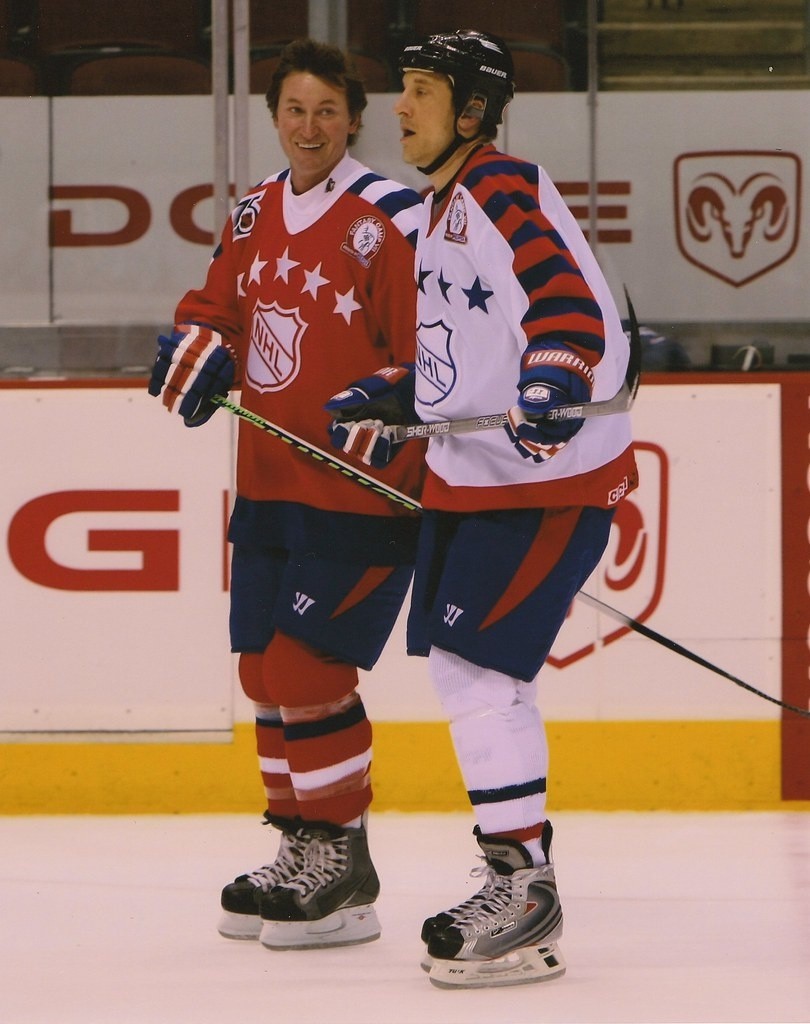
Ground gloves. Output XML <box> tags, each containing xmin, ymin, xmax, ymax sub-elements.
<box><xmin>323</xmin><ymin>363</ymin><xmax>422</xmax><ymax>469</ymax></box>
<box><xmin>504</xmin><ymin>341</ymin><xmax>594</xmax><ymax>463</ymax></box>
<box><xmin>147</xmin><ymin>321</ymin><xmax>236</xmax><ymax>427</ymax></box>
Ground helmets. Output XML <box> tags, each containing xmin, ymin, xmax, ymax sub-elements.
<box><xmin>398</xmin><ymin>28</ymin><xmax>515</xmax><ymax>142</ymax></box>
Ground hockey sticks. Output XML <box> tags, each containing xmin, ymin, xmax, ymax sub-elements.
<box><xmin>216</xmin><ymin>391</ymin><xmax>810</xmax><ymax>717</ymax></box>
<box><xmin>382</xmin><ymin>279</ymin><xmax>643</xmax><ymax>445</ymax></box>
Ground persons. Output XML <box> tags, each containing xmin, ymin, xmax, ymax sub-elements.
<box><xmin>149</xmin><ymin>42</ymin><xmax>422</xmax><ymax>950</ymax></box>
<box><xmin>322</xmin><ymin>28</ymin><xmax>639</xmax><ymax>987</ymax></box>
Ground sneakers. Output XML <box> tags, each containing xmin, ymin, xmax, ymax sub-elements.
<box><xmin>427</xmin><ymin>826</ymin><xmax>566</xmax><ymax>985</ymax></box>
<box><xmin>420</xmin><ymin>818</ymin><xmax>552</xmax><ymax>971</ymax></box>
<box><xmin>215</xmin><ymin>809</ymin><xmax>304</xmax><ymax>935</ymax></box>
<box><xmin>260</xmin><ymin>814</ymin><xmax>381</xmax><ymax>946</ymax></box>
